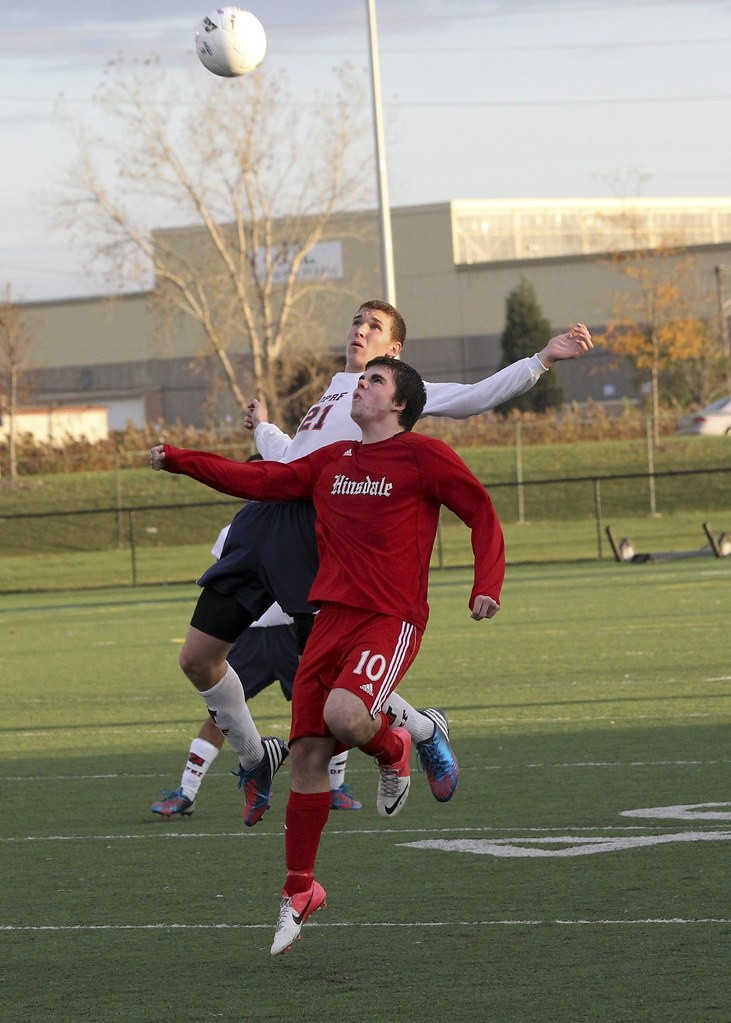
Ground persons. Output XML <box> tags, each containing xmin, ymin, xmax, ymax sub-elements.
<box><xmin>147</xmin><ymin>354</ymin><xmax>504</xmax><ymax>953</ymax></box>
<box><xmin>148</xmin><ymin>295</ymin><xmax>594</xmax><ymax>825</ymax></box>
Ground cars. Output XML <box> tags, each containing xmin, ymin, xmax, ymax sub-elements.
<box><xmin>679</xmin><ymin>397</ymin><xmax>731</xmax><ymax>437</ymax></box>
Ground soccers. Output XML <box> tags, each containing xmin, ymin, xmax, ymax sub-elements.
<box><xmin>195</xmin><ymin>6</ymin><xmax>267</xmax><ymax>79</ymax></box>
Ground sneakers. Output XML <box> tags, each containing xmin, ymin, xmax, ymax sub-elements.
<box><xmin>415</xmin><ymin>707</ymin><xmax>458</xmax><ymax>802</ymax></box>
<box><xmin>270</xmin><ymin>880</ymin><xmax>327</xmax><ymax>956</ymax></box>
<box><xmin>373</xmin><ymin>727</ymin><xmax>411</xmax><ymax>818</ymax></box>
<box><xmin>230</xmin><ymin>736</ymin><xmax>289</xmax><ymax>827</ymax></box>
<box><xmin>151</xmin><ymin>787</ymin><xmax>196</xmax><ymax>818</ymax></box>
<box><xmin>329</xmin><ymin>784</ymin><xmax>362</xmax><ymax>810</ymax></box>
